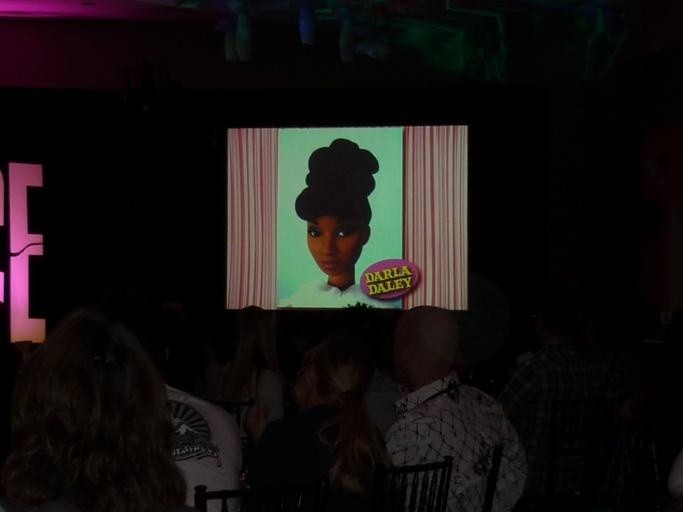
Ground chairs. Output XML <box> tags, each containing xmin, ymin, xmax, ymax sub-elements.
<box><xmin>194</xmin><ymin>398</ymin><xmax>683</xmax><ymax>512</ymax></box>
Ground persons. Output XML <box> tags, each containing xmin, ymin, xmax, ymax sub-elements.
<box><xmin>1</xmin><ymin>306</ymin><xmax>682</xmax><ymax>511</ymax></box>
<box><xmin>278</xmin><ymin>138</ymin><xmax>393</xmax><ymax>308</ymax></box>
